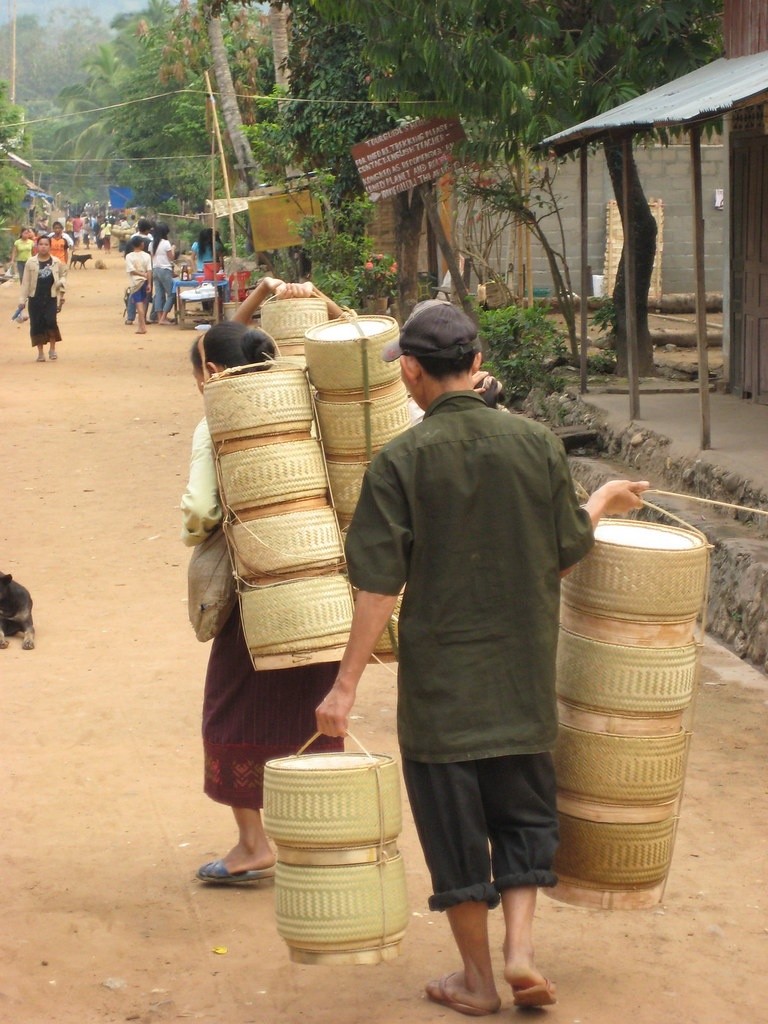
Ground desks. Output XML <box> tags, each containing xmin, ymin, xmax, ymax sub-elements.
<box><xmin>171</xmin><ymin>275</ymin><xmax>229</xmax><ymax>325</ymax></box>
<box><xmin>178</xmin><ymin>291</ymin><xmax>220</xmax><ymax>328</ymax></box>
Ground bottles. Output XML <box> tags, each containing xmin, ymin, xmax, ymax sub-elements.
<box><xmin>181</xmin><ymin>265</ymin><xmax>188</xmax><ymax>281</ymax></box>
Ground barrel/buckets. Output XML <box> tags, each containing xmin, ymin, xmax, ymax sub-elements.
<box><xmin>223</xmin><ymin>303</ymin><xmax>242</xmax><ymax>322</ymax></box>
<box><xmin>201</xmin><ymin>280</ymin><xmax>231</xmax><ymax>314</ymax></box>
<box><xmin>592</xmin><ymin>274</ymin><xmax>604</xmax><ymax>298</ymax></box>
<box><xmin>203</xmin><ymin>261</ymin><xmax>219</xmax><ymax>281</ymax></box>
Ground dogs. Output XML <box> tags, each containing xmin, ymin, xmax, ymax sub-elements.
<box><xmin>69</xmin><ymin>249</ymin><xmax>92</xmax><ymax>270</ymax></box>
<box><xmin>0</xmin><ymin>572</ymin><xmax>37</xmax><ymax>651</ymax></box>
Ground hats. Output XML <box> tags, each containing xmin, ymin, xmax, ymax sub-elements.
<box><xmin>380</xmin><ymin>296</ymin><xmax>482</xmax><ymax>359</ymax></box>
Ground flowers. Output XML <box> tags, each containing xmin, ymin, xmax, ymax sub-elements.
<box><xmin>358</xmin><ymin>252</ymin><xmax>396</xmax><ymax>301</ymax></box>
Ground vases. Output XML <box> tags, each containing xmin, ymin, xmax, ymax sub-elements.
<box><xmin>363</xmin><ymin>294</ymin><xmax>389</xmax><ymax>316</ymax></box>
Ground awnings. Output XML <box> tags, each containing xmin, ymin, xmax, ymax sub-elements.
<box><xmin>539</xmin><ymin>51</ymin><xmax>768</xmax><ymax>450</ymax></box>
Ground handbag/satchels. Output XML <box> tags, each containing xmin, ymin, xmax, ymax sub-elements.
<box><xmin>5</xmin><ymin>265</ymin><xmax>12</xmax><ymax>278</ymax></box>
<box><xmin>186</xmin><ymin>522</ymin><xmax>241</xmax><ymax>643</ymax></box>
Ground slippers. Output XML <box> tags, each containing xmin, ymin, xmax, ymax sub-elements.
<box><xmin>125</xmin><ymin>320</ymin><xmax>133</xmax><ymax>325</ymax></box>
<box><xmin>426</xmin><ymin>970</ymin><xmax>493</xmax><ymax>1015</ymax></box>
<box><xmin>511</xmin><ymin>971</ymin><xmax>556</xmax><ymax>1009</ymax></box>
<box><xmin>37</xmin><ymin>355</ymin><xmax>46</xmax><ymax>361</ymax></box>
<box><xmin>196</xmin><ymin>858</ymin><xmax>276</xmax><ymax>883</ymax></box>
<box><xmin>49</xmin><ymin>350</ymin><xmax>57</xmax><ymax>360</ymax></box>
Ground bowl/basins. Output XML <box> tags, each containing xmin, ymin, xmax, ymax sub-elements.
<box><xmin>195</xmin><ymin>276</ymin><xmax>204</xmax><ymax>281</ymax></box>
<box><xmin>216</xmin><ymin>273</ymin><xmax>224</xmax><ymax>280</ymax></box>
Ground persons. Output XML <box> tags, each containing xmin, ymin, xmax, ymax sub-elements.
<box><xmin>191</xmin><ymin>228</ymin><xmax>224</xmax><ymax>312</ymax></box>
<box><xmin>181</xmin><ymin>322</ymin><xmax>344</xmax><ymax>884</ymax></box>
<box><xmin>18</xmin><ymin>236</ymin><xmax>66</xmax><ymax>361</ymax></box>
<box><xmin>407</xmin><ymin>370</ymin><xmax>504</xmax><ymax>426</ymax></box>
<box><xmin>124</xmin><ymin>220</ymin><xmax>176</xmax><ymax>334</ymax></box>
<box><xmin>11</xmin><ymin>212</ymin><xmax>69</xmax><ymax>313</ymax></box>
<box><xmin>66</xmin><ymin>201</ymin><xmax>136</xmax><ymax>255</ymax></box>
<box><xmin>232</xmin><ymin>276</ymin><xmax>342</xmax><ymax>319</ymax></box>
<box><xmin>316</xmin><ymin>303</ymin><xmax>650</xmax><ymax>1017</ymax></box>
